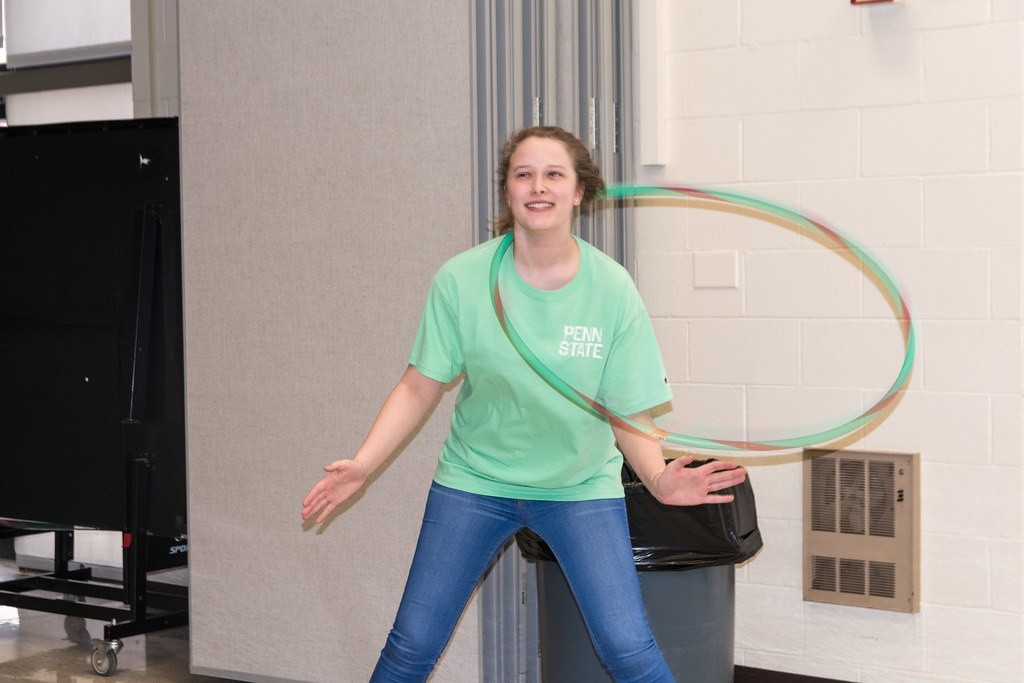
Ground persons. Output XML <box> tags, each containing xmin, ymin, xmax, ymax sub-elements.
<box><xmin>300</xmin><ymin>122</ymin><xmax>748</xmax><ymax>683</ymax></box>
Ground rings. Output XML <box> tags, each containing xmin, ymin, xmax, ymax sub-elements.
<box><xmin>707</xmin><ymin>484</ymin><xmax>711</xmax><ymax>493</ymax></box>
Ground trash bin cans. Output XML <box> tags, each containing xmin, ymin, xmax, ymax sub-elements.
<box><xmin>515</xmin><ymin>458</ymin><xmax>763</xmax><ymax>683</ymax></box>
